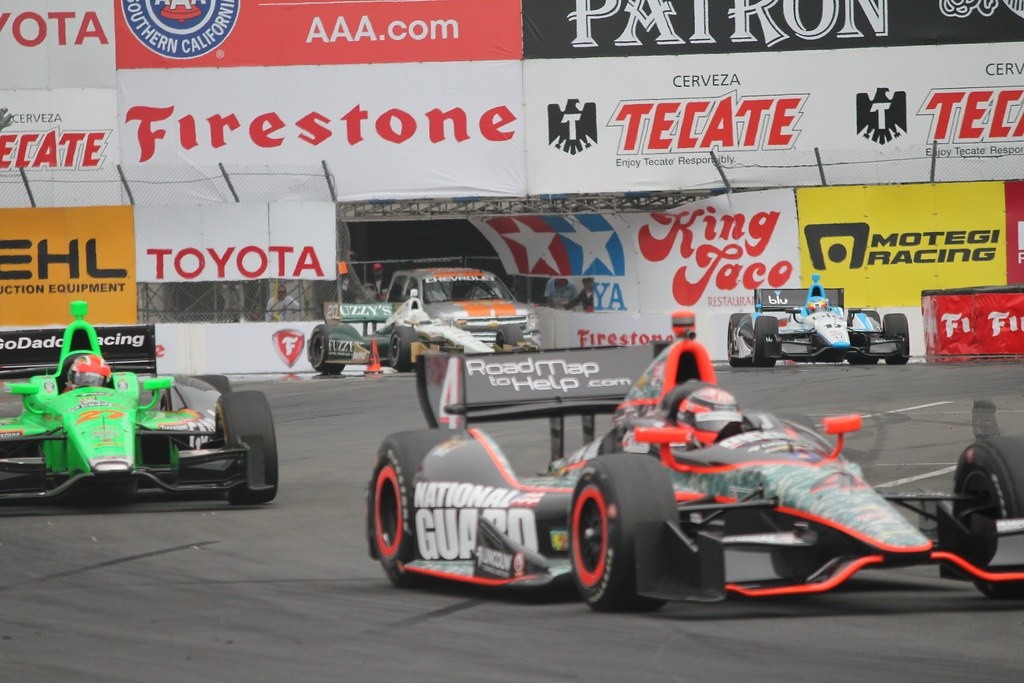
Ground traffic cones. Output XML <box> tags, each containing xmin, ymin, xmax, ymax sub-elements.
<box><xmin>363</xmin><ymin>337</ymin><xmax>388</xmax><ymax>376</ymax></box>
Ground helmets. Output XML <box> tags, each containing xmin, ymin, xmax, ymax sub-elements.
<box><xmin>806</xmin><ymin>295</ymin><xmax>828</xmax><ymax>316</ymax></box>
<box><xmin>66</xmin><ymin>355</ymin><xmax>111</xmax><ymax>391</ymax></box>
<box><xmin>676</xmin><ymin>388</ymin><xmax>742</xmax><ymax>450</ymax></box>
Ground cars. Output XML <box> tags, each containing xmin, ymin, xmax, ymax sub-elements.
<box><xmin>382</xmin><ymin>267</ymin><xmax>540</xmax><ymax>358</ymax></box>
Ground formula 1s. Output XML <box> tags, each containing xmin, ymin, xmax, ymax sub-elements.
<box><xmin>727</xmin><ymin>271</ymin><xmax>912</xmax><ymax>367</ymax></box>
<box><xmin>0</xmin><ymin>300</ymin><xmax>279</xmax><ymax>507</ymax></box>
<box><xmin>306</xmin><ymin>290</ymin><xmax>494</xmax><ymax>379</ymax></box>
<box><xmin>366</xmin><ymin>314</ymin><xmax>1023</xmax><ymax>617</ymax></box>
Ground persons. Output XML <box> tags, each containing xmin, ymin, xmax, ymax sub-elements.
<box><xmin>676</xmin><ymin>387</ymin><xmax>738</xmax><ymax>448</ymax></box>
<box><xmin>265</xmin><ymin>286</ymin><xmax>302</xmax><ymax>322</ymax></box>
<box><xmin>565</xmin><ymin>277</ymin><xmax>594</xmax><ymax>311</ymax></box>
<box><xmin>544</xmin><ymin>277</ymin><xmax>576</xmax><ymax>310</ymax></box>
<box><xmin>65</xmin><ymin>354</ymin><xmax>111</xmax><ymax>392</ymax></box>
<box><xmin>808</xmin><ymin>296</ymin><xmax>827</xmax><ymax>313</ymax></box>
<box><xmin>370</xmin><ymin>263</ymin><xmax>389</xmax><ymax>302</ymax></box>
